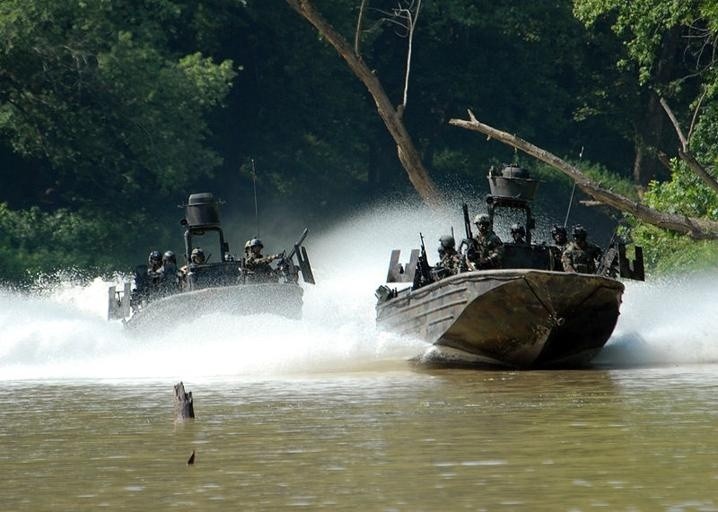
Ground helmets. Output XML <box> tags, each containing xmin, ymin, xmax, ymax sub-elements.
<box><xmin>250</xmin><ymin>239</ymin><xmax>263</xmax><ymax>248</ymax></box>
<box><xmin>245</xmin><ymin>239</ymin><xmax>251</xmax><ymax>248</ymax></box>
<box><xmin>438</xmin><ymin>235</ymin><xmax>455</xmax><ymax>249</ymax></box>
<box><xmin>510</xmin><ymin>223</ymin><xmax>526</xmax><ymax>234</ymax></box>
<box><xmin>472</xmin><ymin>213</ymin><xmax>491</xmax><ymax>225</ymax></box>
<box><xmin>149</xmin><ymin>250</ymin><xmax>162</xmax><ymax>260</ymax></box>
<box><xmin>572</xmin><ymin>226</ymin><xmax>587</xmax><ymax>235</ymax></box>
<box><xmin>161</xmin><ymin>250</ymin><xmax>177</xmax><ymax>262</ymax></box>
<box><xmin>551</xmin><ymin>225</ymin><xmax>566</xmax><ymax>236</ymax></box>
<box><xmin>191</xmin><ymin>248</ymin><xmax>205</xmax><ymax>258</ymax></box>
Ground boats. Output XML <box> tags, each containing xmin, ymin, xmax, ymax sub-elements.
<box><xmin>107</xmin><ymin>159</ymin><xmax>316</xmax><ymax>369</ymax></box>
<box><xmin>372</xmin><ymin>146</ymin><xmax>646</xmax><ymax>374</ymax></box>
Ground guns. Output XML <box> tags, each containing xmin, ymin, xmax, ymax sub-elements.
<box><xmin>451</xmin><ymin>226</ymin><xmax>456</xmax><ymax>249</ymax></box>
<box><xmin>418</xmin><ymin>232</ymin><xmax>430</xmax><ymax>276</ymax></box>
<box><xmin>462</xmin><ymin>202</ymin><xmax>474</xmax><ymax>240</ymax></box>
<box><xmin>596</xmin><ymin>232</ymin><xmax>617</xmax><ymax>274</ymax></box>
<box><xmin>278</xmin><ymin>227</ymin><xmax>309</xmax><ymax>267</ymax></box>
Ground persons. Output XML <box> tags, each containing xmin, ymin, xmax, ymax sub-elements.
<box><xmin>148</xmin><ymin>237</ymin><xmax>283</xmax><ymax>295</ymax></box>
<box><xmin>430</xmin><ymin>213</ymin><xmax>606</xmax><ymax>281</ymax></box>
